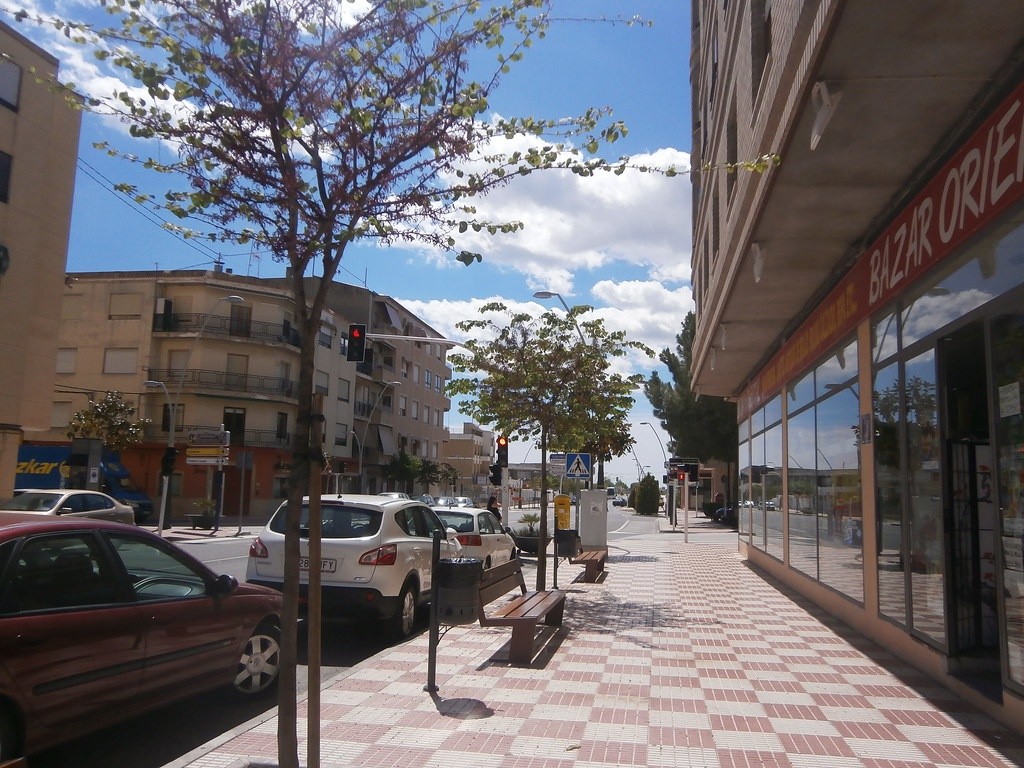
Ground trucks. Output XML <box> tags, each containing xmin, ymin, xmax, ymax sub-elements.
<box><xmin>15</xmin><ymin>444</ymin><xmax>154</xmax><ymax>524</ymax></box>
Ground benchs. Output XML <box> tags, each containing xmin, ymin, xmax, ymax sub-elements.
<box><xmin>568</xmin><ymin>536</ymin><xmax>607</xmax><ymax>583</ymax></box>
<box><xmin>477</xmin><ymin>558</ymin><xmax>566</xmax><ymax>666</ymax></box>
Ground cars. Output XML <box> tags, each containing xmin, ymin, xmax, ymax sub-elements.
<box><xmin>407</xmin><ymin>505</ymin><xmax>516</xmax><ymax>583</ymax></box>
<box><xmin>453</xmin><ymin>496</ymin><xmax>474</xmax><ymax>508</ymax></box>
<box><xmin>739</xmin><ymin>500</ymin><xmax>754</xmax><ymax>508</ymax></box>
<box><xmin>758</xmin><ymin>501</ymin><xmax>775</xmax><ymax>511</ymax></box>
<box><xmin>408</xmin><ymin>493</ymin><xmax>437</xmax><ymax>507</ymax></box>
<box><xmin>375</xmin><ymin>492</ymin><xmax>411</xmax><ymax>500</ymax></box>
<box><xmin>1</xmin><ymin>511</ymin><xmax>284</xmax><ymax>768</ymax></box>
<box><xmin>0</xmin><ymin>488</ymin><xmax>135</xmax><ymax>547</ymax></box>
<box><xmin>613</xmin><ymin>497</ymin><xmax>628</xmax><ymax>507</ymax></box>
<box><xmin>432</xmin><ymin>496</ymin><xmax>459</xmax><ymax>507</ymax></box>
<box><xmin>246</xmin><ymin>492</ymin><xmax>462</xmax><ymax>641</ymax></box>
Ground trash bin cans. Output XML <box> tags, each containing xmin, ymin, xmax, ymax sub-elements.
<box><xmin>558</xmin><ymin>530</ymin><xmax>578</xmax><ymax>557</ymax></box>
<box><xmin>438</xmin><ymin>558</ymin><xmax>483</xmax><ymax>625</ymax></box>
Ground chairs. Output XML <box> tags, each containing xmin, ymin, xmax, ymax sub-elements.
<box><xmin>54</xmin><ymin>554</ymin><xmax>93</xmax><ymax>595</ymax></box>
<box><xmin>458</xmin><ymin>524</ymin><xmax>473</xmax><ymax>532</ymax></box>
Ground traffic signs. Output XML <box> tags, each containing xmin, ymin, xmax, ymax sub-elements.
<box><xmin>185</xmin><ymin>446</ymin><xmax>230</xmax><ymax>456</ymax></box>
<box><xmin>186</xmin><ymin>456</ymin><xmax>230</xmax><ymax>465</ymax></box>
<box><xmin>187</xmin><ymin>430</ymin><xmax>230</xmax><ymax>447</ymax></box>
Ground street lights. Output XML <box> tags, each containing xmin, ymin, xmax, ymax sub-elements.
<box><xmin>342</xmin><ymin>381</ymin><xmax>402</xmax><ymax>475</ymax></box>
<box><xmin>141</xmin><ymin>295</ymin><xmax>246</xmax><ymax>537</ymax></box>
<box><xmin>632</xmin><ymin>458</ymin><xmax>651</xmax><ymax>482</ymax></box>
<box><xmin>639</xmin><ymin>422</ymin><xmax>668</xmax><ymax>463</ymax></box>
<box><xmin>532</xmin><ymin>291</ymin><xmax>604</xmax><ymax>490</ymax></box>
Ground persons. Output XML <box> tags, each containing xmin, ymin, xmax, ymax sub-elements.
<box><xmin>486</xmin><ymin>496</ymin><xmax>502</xmax><ymax>524</ymax></box>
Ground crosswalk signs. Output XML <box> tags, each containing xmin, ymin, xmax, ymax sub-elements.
<box><xmin>566</xmin><ymin>452</ymin><xmax>591</xmax><ymax>479</ymax></box>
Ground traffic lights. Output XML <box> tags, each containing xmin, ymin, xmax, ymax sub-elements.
<box><xmin>678</xmin><ymin>470</ymin><xmax>685</xmax><ymax>486</ymax></box>
<box><xmin>489</xmin><ymin>465</ymin><xmax>501</xmax><ymax>485</ymax></box>
<box><xmin>495</xmin><ymin>435</ymin><xmax>508</xmax><ymax>468</ymax></box>
<box><xmin>346</xmin><ymin>323</ymin><xmax>367</xmax><ymax>362</ymax></box>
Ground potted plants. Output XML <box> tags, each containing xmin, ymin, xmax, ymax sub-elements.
<box><xmin>514</xmin><ymin>512</ymin><xmax>554</xmax><ymax>553</ymax></box>
<box><xmin>186</xmin><ymin>496</ymin><xmax>225</xmax><ymax>531</ymax></box>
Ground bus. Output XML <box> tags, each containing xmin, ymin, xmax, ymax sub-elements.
<box><xmin>606</xmin><ymin>486</ymin><xmax>615</xmax><ymax>499</ymax></box>
